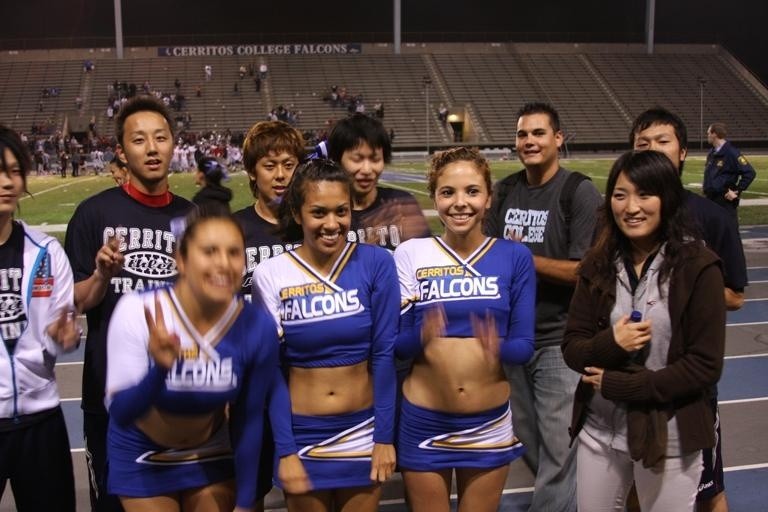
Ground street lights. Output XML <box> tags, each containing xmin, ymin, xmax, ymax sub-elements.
<box><xmin>421</xmin><ymin>75</ymin><xmax>433</xmax><ymax>154</ymax></box>
<box><xmin>698</xmin><ymin>78</ymin><xmax>707</xmax><ymax>151</ymax></box>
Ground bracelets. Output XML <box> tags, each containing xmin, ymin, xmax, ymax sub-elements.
<box><xmin>91</xmin><ymin>268</ymin><xmax>110</xmax><ymax>284</ymax></box>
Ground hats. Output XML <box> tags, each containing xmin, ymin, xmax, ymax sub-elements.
<box><xmin>194</xmin><ymin>150</ymin><xmax>222</xmax><ymax>182</ymax></box>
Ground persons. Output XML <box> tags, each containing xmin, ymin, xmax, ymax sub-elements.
<box><xmin>393</xmin><ymin>145</ymin><xmax>537</xmax><ymax>511</ymax></box>
<box><xmin>702</xmin><ymin>122</ymin><xmax>756</xmax><ymax>287</ymax></box>
<box><xmin>251</xmin><ymin>156</ymin><xmax>401</xmax><ymax>512</ymax></box>
<box><xmin>109</xmin><ymin>155</ymin><xmax>130</xmax><ymax>186</ymax></box>
<box><xmin>326</xmin><ymin>111</ymin><xmax>431</xmax><ymax>474</ymax></box>
<box><xmin>65</xmin><ymin>95</ymin><xmax>201</xmax><ymax>511</ymax></box>
<box><xmin>560</xmin><ymin>149</ymin><xmax>727</xmax><ymax>511</ymax></box>
<box><xmin>0</xmin><ymin>125</ymin><xmax>81</xmax><ymax>512</ymax></box>
<box><xmin>190</xmin><ymin>151</ymin><xmax>233</xmax><ymax>209</ymax></box>
<box><xmin>19</xmin><ymin>60</ymin><xmax>384</xmax><ymax>174</ymax></box>
<box><xmin>437</xmin><ymin>102</ymin><xmax>449</xmax><ymax>129</ymax></box>
<box><xmin>482</xmin><ymin>100</ymin><xmax>606</xmax><ymax>510</ymax></box>
<box><xmin>628</xmin><ymin>103</ymin><xmax>748</xmax><ymax>511</ymax></box>
<box><xmin>20</xmin><ymin>133</ymin><xmax>36</xmax><ymax>200</ymax></box>
<box><xmin>103</xmin><ymin>207</ymin><xmax>278</xmax><ymax>512</ymax></box>
<box><xmin>231</xmin><ymin>119</ymin><xmax>307</xmax><ymax>511</ymax></box>
